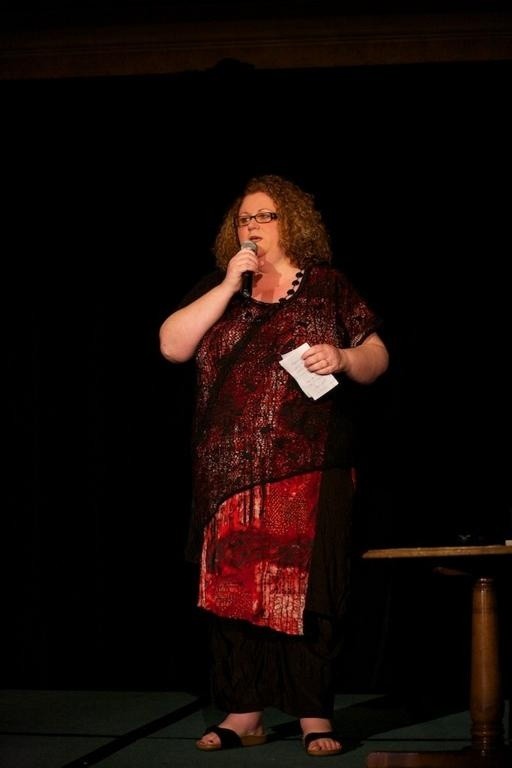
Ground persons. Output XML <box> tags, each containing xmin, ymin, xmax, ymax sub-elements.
<box><xmin>158</xmin><ymin>175</ymin><xmax>388</xmax><ymax>755</ymax></box>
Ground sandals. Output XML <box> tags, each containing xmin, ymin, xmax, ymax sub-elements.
<box><xmin>195</xmin><ymin>725</ymin><xmax>267</xmax><ymax>750</ymax></box>
<box><xmin>303</xmin><ymin>732</ymin><xmax>343</xmax><ymax>756</ymax></box>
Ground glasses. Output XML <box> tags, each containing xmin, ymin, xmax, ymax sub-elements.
<box><xmin>234</xmin><ymin>212</ymin><xmax>277</xmax><ymax>227</ymax></box>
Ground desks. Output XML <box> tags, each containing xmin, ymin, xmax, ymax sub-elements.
<box><xmin>360</xmin><ymin>539</ymin><xmax>511</xmax><ymax>768</ymax></box>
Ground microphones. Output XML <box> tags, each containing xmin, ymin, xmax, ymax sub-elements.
<box><xmin>240</xmin><ymin>240</ymin><xmax>257</xmax><ymax>299</ymax></box>
